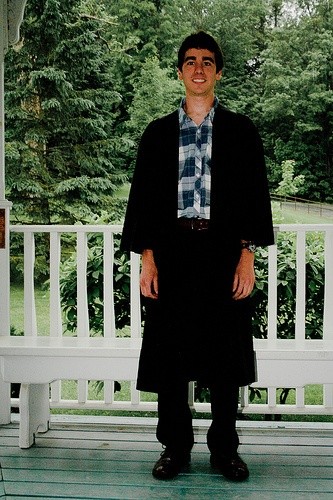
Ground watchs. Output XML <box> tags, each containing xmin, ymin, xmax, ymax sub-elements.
<box><xmin>242</xmin><ymin>241</ymin><xmax>257</xmax><ymax>253</ymax></box>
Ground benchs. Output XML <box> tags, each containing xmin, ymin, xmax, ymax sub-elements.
<box><xmin>0</xmin><ymin>334</ymin><xmax>332</xmax><ymax>449</ymax></box>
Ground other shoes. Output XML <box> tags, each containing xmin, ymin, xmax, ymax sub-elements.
<box><xmin>152</xmin><ymin>444</ymin><xmax>192</xmax><ymax>481</ymax></box>
<box><xmin>209</xmin><ymin>447</ymin><xmax>249</xmax><ymax>482</ymax></box>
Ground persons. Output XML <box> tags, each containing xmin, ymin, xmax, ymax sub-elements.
<box><xmin>120</xmin><ymin>31</ymin><xmax>276</xmax><ymax>482</ymax></box>
